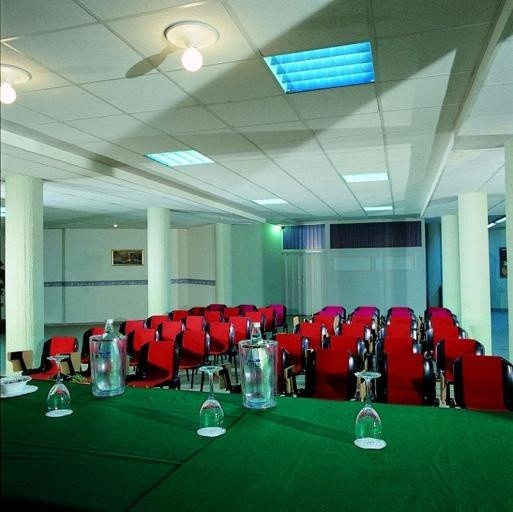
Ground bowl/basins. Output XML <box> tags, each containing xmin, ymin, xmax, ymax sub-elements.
<box><xmin>0</xmin><ymin>376</ymin><xmax>30</xmax><ymax>394</ymax></box>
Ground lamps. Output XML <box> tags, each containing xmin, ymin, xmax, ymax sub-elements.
<box><xmin>164</xmin><ymin>20</ymin><xmax>220</xmax><ymax>72</ymax></box>
<box><xmin>0</xmin><ymin>64</ymin><xmax>31</xmax><ymax>105</ymax></box>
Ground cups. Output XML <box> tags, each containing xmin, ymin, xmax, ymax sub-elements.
<box><xmin>237</xmin><ymin>339</ymin><xmax>277</xmax><ymax>410</ymax></box>
<box><xmin>89</xmin><ymin>334</ymin><xmax>127</xmax><ymax>397</ymax></box>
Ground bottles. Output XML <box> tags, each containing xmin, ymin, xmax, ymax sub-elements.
<box><xmin>96</xmin><ymin>319</ymin><xmax>121</xmax><ymax>391</ymax></box>
<box><xmin>243</xmin><ymin>320</ymin><xmax>271</xmax><ymax>403</ymax></box>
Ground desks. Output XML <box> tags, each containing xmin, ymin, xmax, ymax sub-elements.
<box><xmin>0</xmin><ymin>374</ymin><xmax>512</xmax><ymax>511</ymax></box>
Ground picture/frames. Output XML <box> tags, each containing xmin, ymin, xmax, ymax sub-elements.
<box><xmin>499</xmin><ymin>247</ymin><xmax>507</xmax><ymax>280</ymax></box>
<box><xmin>111</xmin><ymin>248</ymin><xmax>144</xmax><ymax>266</ymax></box>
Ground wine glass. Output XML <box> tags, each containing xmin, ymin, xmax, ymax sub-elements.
<box><xmin>196</xmin><ymin>364</ymin><xmax>226</xmax><ymax>439</ymax></box>
<box><xmin>43</xmin><ymin>355</ymin><xmax>76</xmax><ymax>418</ymax></box>
<box><xmin>354</xmin><ymin>370</ymin><xmax>389</xmax><ymax>450</ymax></box>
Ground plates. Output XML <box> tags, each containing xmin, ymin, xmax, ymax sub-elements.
<box><xmin>0</xmin><ymin>382</ymin><xmax>39</xmax><ymax>398</ymax></box>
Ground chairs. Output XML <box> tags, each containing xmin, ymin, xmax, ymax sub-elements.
<box><xmin>294</xmin><ymin>306</ymin><xmax>485</xmax><ymax>402</ymax></box>
<box><xmin>80</xmin><ymin>303</ymin><xmax>288</xmax><ymax>389</ymax></box>
<box><xmin>271</xmin><ymin>332</ymin><xmax>309</xmax><ymax>396</ymax></box>
<box><xmin>371</xmin><ymin>351</ymin><xmax>436</xmax><ymax>406</ymax></box>
<box><xmin>22</xmin><ymin>336</ymin><xmax>79</xmax><ymax>381</ymax></box>
<box><xmin>446</xmin><ymin>355</ymin><xmax>512</xmax><ymax>410</ymax></box>
